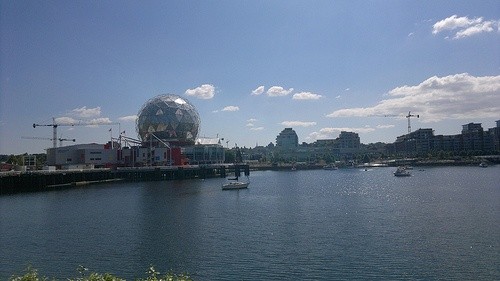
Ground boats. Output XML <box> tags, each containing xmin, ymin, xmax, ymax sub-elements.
<box><xmin>322</xmin><ymin>163</ymin><xmax>338</xmax><ymax>170</ymax></box>
<box><xmin>479</xmin><ymin>162</ymin><xmax>488</xmax><ymax>167</ymax></box>
<box><xmin>222</xmin><ymin>181</ymin><xmax>250</xmax><ymax>190</ymax></box>
<box><xmin>393</xmin><ymin>166</ymin><xmax>410</xmax><ymax>176</ymax></box>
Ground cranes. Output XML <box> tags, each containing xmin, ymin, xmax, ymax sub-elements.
<box><xmin>20</xmin><ymin>122</ymin><xmax>76</xmax><ymax>147</ymax></box>
<box><xmin>383</xmin><ymin>111</ymin><xmax>419</xmax><ymax>133</ymax></box>
